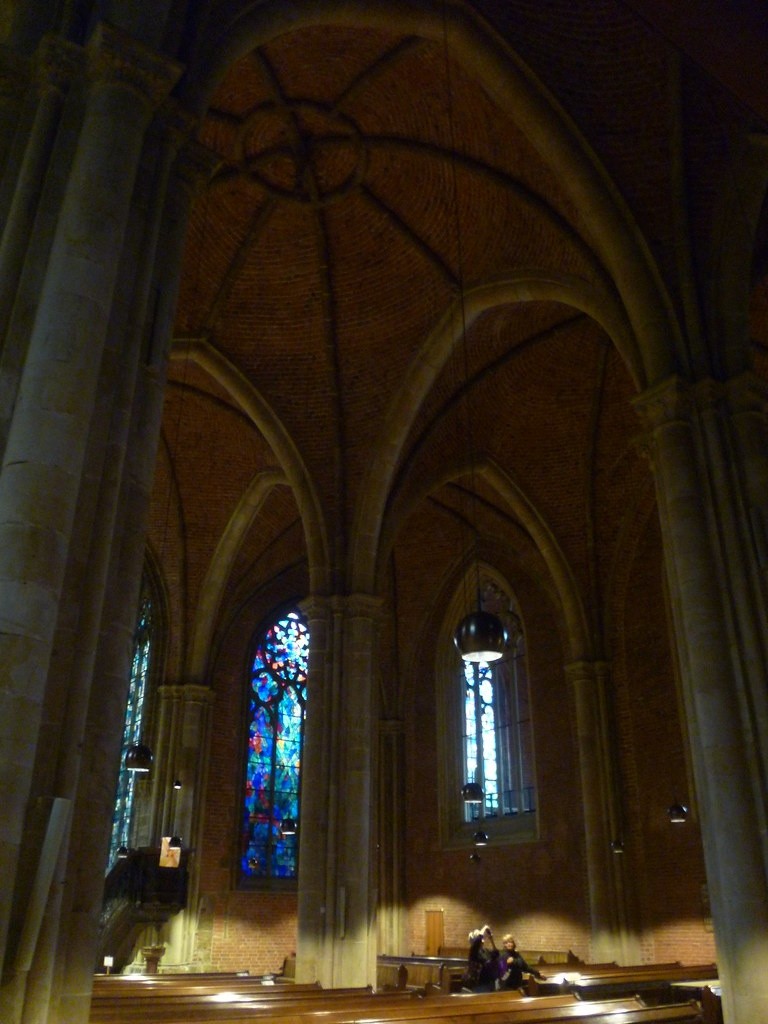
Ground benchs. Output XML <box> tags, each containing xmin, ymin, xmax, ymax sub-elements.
<box><xmin>88</xmin><ymin>945</ymin><xmax>723</xmax><ymax>1024</ymax></box>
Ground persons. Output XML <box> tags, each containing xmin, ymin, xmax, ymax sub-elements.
<box><xmin>461</xmin><ymin>924</ymin><xmax>546</xmax><ymax>996</ymax></box>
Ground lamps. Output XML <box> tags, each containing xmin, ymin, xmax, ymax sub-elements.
<box><xmin>280</xmin><ymin>818</ymin><xmax>297</xmax><ymax>834</ymax></box>
<box><xmin>173</xmin><ymin>780</ymin><xmax>182</xmax><ymax>790</ymax></box>
<box><xmin>443</xmin><ymin>18</ymin><xmax>484</xmax><ymax>803</ymax></box>
<box><xmin>470</xmin><ymin>852</ymin><xmax>483</xmax><ymax>864</ymax></box>
<box><xmin>667</xmin><ymin>803</ymin><xmax>688</xmax><ymax>823</ymax></box>
<box><xmin>611</xmin><ymin>839</ymin><xmax>624</xmax><ymax>853</ymax></box>
<box><xmin>125</xmin><ymin>745</ymin><xmax>154</xmax><ymax>772</ymax></box>
<box><xmin>168</xmin><ymin>836</ymin><xmax>182</xmax><ymax>849</ymax></box>
<box><xmin>442</xmin><ymin>0</ymin><xmax>506</xmax><ymax>662</ymax></box>
<box><xmin>117</xmin><ymin>847</ymin><xmax>128</xmax><ymax>858</ymax></box>
<box><xmin>472</xmin><ymin>831</ymin><xmax>489</xmax><ymax>845</ymax></box>
